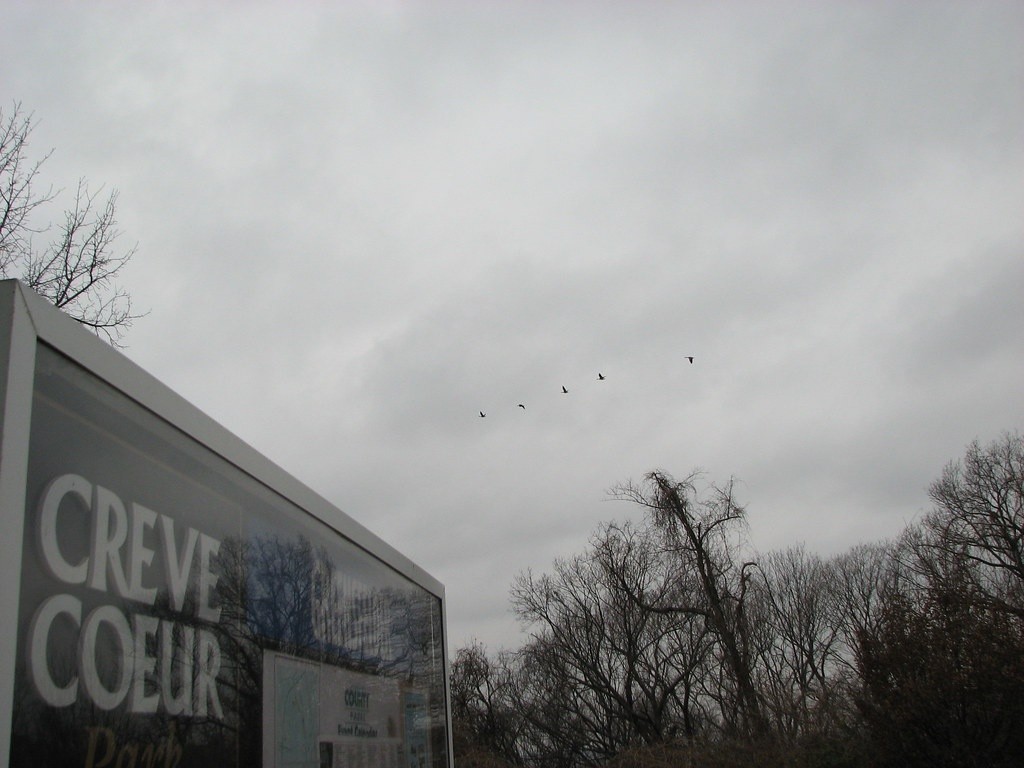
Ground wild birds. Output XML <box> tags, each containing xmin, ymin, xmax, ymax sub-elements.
<box><xmin>560</xmin><ymin>386</ymin><xmax>568</xmax><ymax>393</ymax></box>
<box><xmin>517</xmin><ymin>404</ymin><xmax>525</xmax><ymax>409</ymax></box>
<box><xmin>596</xmin><ymin>373</ymin><xmax>606</xmax><ymax>380</ymax></box>
<box><xmin>684</xmin><ymin>356</ymin><xmax>694</xmax><ymax>364</ymax></box>
<box><xmin>479</xmin><ymin>411</ymin><xmax>486</xmax><ymax>417</ymax></box>
